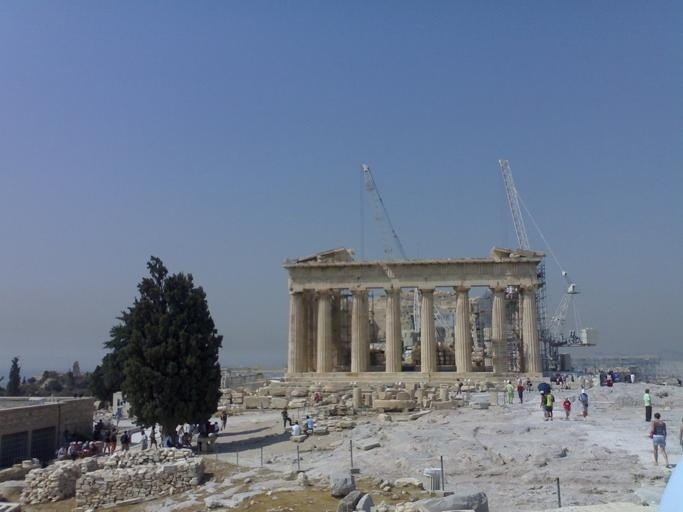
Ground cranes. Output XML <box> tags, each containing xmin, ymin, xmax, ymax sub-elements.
<box><xmin>498</xmin><ymin>158</ymin><xmax>597</xmax><ymax>372</ymax></box>
<box><xmin>361</xmin><ymin>164</ymin><xmax>455</xmax><ymax>371</ymax></box>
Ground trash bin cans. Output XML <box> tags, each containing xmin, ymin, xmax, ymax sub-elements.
<box><xmin>423</xmin><ymin>468</ymin><xmax>443</xmax><ymax>491</ymax></box>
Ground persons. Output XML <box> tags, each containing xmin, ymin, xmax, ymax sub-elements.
<box><xmin>505</xmin><ymin>368</ymin><xmax>637</xmax><ymax>422</ymax></box>
<box><xmin>454</xmin><ymin>378</ymin><xmax>463</xmax><ymax>396</ymax></box>
<box><xmin>280</xmin><ymin>406</ymin><xmax>292</xmax><ymax>429</ymax></box>
<box><xmin>649</xmin><ymin>412</ymin><xmax>670</xmax><ymax>467</ymax></box>
<box><xmin>52</xmin><ymin>410</ymin><xmax>228</xmax><ymax>461</ymax></box>
<box><xmin>313</xmin><ymin>392</ymin><xmax>320</xmax><ymax>403</ymax></box>
<box><xmin>643</xmin><ymin>388</ymin><xmax>652</xmax><ymax>421</ymax></box>
<box><xmin>302</xmin><ymin>415</ymin><xmax>313</xmax><ymax>432</ymax></box>
<box><xmin>289</xmin><ymin>420</ymin><xmax>300</xmax><ymax>435</ymax></box>
